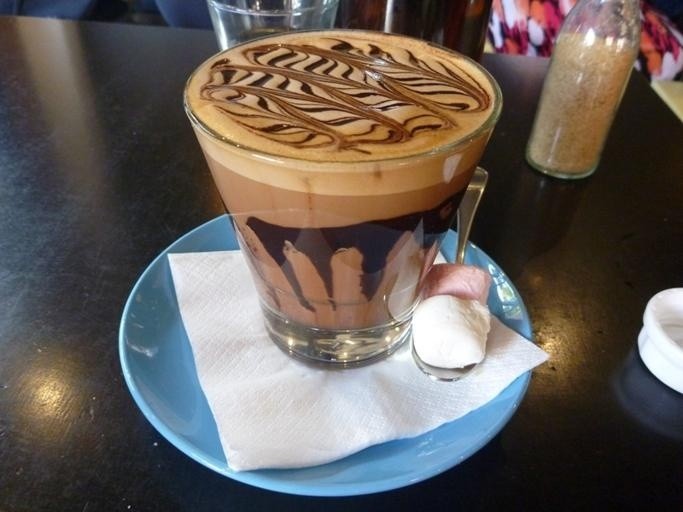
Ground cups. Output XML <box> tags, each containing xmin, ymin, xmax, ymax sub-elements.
<box><xmin>208</xmin><ymin>1</ymin><xmax>338</xmax><ymax>51</ymax></box>
<box><xmin>183</xmin><ymin>29</ymin><xmax>499</xmax><ymax>368</ymax></box>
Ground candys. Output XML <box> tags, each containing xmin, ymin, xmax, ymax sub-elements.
<box><xmin>412</xmin><ymin>263</ymin><xmax>492</xmax><ymax>370</ymax></box>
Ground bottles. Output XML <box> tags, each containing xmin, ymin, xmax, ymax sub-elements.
<box><xmin>522</xmin><ymin>0</ymin><xmax>643</xmax><ymax>180</ymax></box>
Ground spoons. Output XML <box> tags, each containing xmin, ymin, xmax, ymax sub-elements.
<box><xmin>408</xmin><ymin>162</ymin><xmax>508</xmax><ymax>383</ymax></box>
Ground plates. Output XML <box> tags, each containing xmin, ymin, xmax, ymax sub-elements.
<box><xmin>115</xmin><ymin>214</ymin><xmax>538</xmax><ymax>497</ymax></box>
